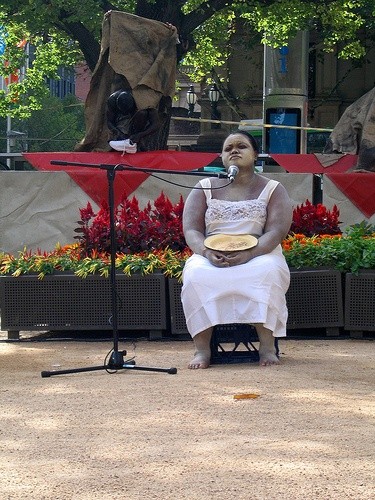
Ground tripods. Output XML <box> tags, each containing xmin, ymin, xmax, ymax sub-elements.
<box><xmin>41</xmin><ymin>160</ymin><xmax>229</xmax><ymax>378</ymax></box>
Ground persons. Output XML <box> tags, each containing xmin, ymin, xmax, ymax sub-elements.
<box><xmin>106</xmin><ymin>87</ymin><xmax>161</xmax><ymax>153</ymax></box>
<box><xmin>181</xmin><ymin>129</ymin><xmax>294</xmax><ymax>368</ymax></box>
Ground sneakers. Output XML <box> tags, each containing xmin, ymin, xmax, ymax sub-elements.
<box><xmin>109</xmin><ymin>139</ymin><xmax>137</xmax><ymax>155</ymax></box>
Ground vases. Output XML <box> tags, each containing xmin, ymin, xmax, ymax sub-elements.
<box><xmin>287</xmin><ymin>266</ymin><xmax>342</xmax><ymax>338</ymax></box>
<box><xmin>0</xmin><ymin>269</ymin><xmax>167</xmax><ymax>341</ymax></box>
<box><xmin>345</xmin><ymin>269</ymin><xmax>375</xmax><ymax>338</ymax></box>
<box><xmin>168</xmin><ymin>272</ymin><xmax>190</xmax><ymax>340</ymax></box>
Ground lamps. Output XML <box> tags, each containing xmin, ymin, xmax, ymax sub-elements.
<box><xmin>187</xmin><ymin>85</ymin><xmax>196</xmax><ymax>114</ymax></box>
<box><xmin>209</xmin><ymin>83</ymin><xmax>220</xmax><ymax>109</ymax></box>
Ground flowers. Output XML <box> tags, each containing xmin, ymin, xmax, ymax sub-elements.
<box><xmin>281</xmin><ymin>199</ymin><xmax>375</xmax><ymax>270</ymax></box>
<box><xmin>0</xmin><ymin>191</ymin><xmax>191</xmax><ymax>282</ymax></box>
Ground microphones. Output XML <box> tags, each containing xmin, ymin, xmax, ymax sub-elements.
<box><xmin>227</xmin><ymin>166</ymin><xmax>240</xmax><ymax>182</ymax></box>
